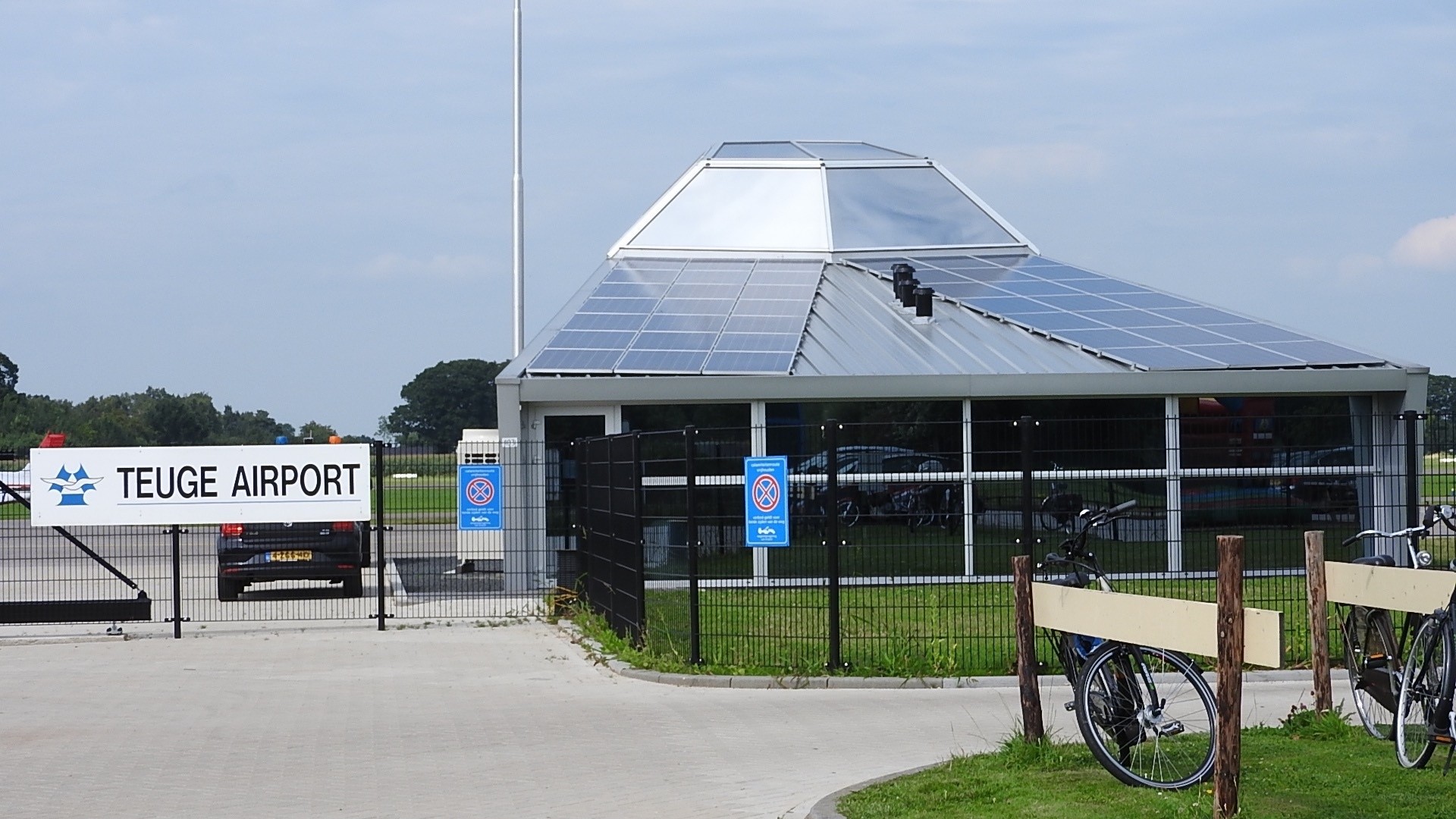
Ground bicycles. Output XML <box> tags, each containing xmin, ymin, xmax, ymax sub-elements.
<box><xmin>1335</xmin><ymin>502</ymin><xmax>1455</xmax><ymax>775</ymax></box>
<box><xmin>1034</xmin><ymin>500</ymin><xmax>1217</xmax><ymax>791</ymax></box>
<box><xmin>787</xmin><ymin>484</ymin><xmax>984</xmax><ymax>534</ymax></box>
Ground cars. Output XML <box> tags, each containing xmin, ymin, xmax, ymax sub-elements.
<box><xmin>779</xmin><ymin>446</ymin><xmax>955</xmax><ymax>495</ymax></box>
<box><xmin>215</xmin><ymin>521</ymin><xmax>365</xmax><ymax>604</ymax></box>
<box><xmin>1183</xmin><ymin>446</ymin><xmax>1354</xmax><ymax>527</ymax></box>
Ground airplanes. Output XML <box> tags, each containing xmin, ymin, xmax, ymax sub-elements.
<box><xmin>0</xmin><ymin>434</ymin><xmax>66</xmax><ymax>503</ymax></box>
